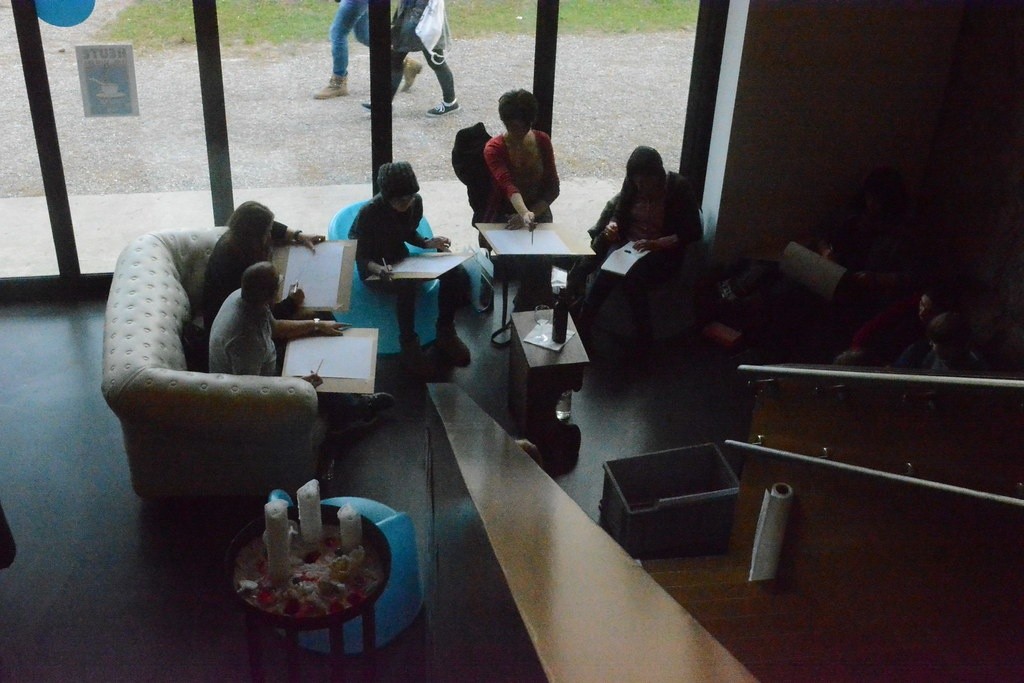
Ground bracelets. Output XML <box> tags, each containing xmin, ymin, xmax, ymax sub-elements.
<box><xmin>313</xmin><ymin>318</ymin><xmax>319</xmax><ymax>333</ymax></box>
<box><xmin>294</xmin><ymin>230</ymin><xmax>302</xmax><ymax>242</ymax></box>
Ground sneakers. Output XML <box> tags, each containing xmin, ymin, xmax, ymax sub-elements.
<box><xmin>426</xmin><ymin>99</ymin><xmax>460</xmax><ymax>117</ymax></box>
<box><xmin>362</xmin><ymin>103</ymin><xmax>372</xmax><ymax>108</ymax></box>
<box><xmin>401</xmin><ymin>58</ymin><xmax>422</xmax><ymax>92</ymax></box>
<box><xmin>329</xmin><ymin>414</ymin><xmax>377</xmax><ymax>435</ymax></box>
<box><xmin>355</xmin><ymin>393</ymin><xmax>395</xmax><ymax>412</ymax></box>
<box><xmin>315</xmin><ymin>76</ymin><xmax>348</xmax><ymax>99</ymax></box>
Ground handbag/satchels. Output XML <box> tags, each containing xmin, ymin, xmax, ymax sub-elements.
<box><xmin>491</xmin><ymin>276</ymin><xmax>585</xmax><ymax>348</ymax></box>
<box><xmin>415</xmin><ymin>0</ymin><xmax>453</xmax><ymax>65</ymax></box>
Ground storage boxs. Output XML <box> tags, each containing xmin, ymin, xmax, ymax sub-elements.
<box><xmin>599</xmin><ymin>440</ymin><xmax>742</xmax><ymax>560</ymax></box>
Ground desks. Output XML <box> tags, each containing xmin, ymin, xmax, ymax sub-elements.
<box><xmin>507</xmin><ymin>308</ymin><xmax>591</xmax><ymax>444</ymax></box>
<box><xmin>475</xmin><ymin>221</ymin><xmax>598</xmax><ymax>347</ymax></box>
<box><xmin>218</xmin><ymin>503</ymin><xmax>392</xmax><ymax>683</ymax></box>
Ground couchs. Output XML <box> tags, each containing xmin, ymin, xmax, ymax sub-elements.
<box><xmin>101</xmin><ymin>223</ymin><xmax>326</xmax><ymax>504</ymax></box>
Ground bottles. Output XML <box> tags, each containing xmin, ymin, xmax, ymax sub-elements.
<box><xmin>552</xmin><ymin>286</ymin><xmax>568</xmax><ymax>344</ymax></box>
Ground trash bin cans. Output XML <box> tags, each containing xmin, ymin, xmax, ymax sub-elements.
<box><xmin>600</xmin><ymin>443</ymin><xmax>739</xmax><ymax>558</ymax></box>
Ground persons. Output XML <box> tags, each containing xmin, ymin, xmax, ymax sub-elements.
<box><xmin>203</xmin><ymin>201</ymin><xmax>397</xmax><ymax>426</ymax></box>
<box><xmin>360</xmin><ymin>0</ymin><xmax>461</xmax><ymax>115</ymax></box>
<box><xmin>312</xmin><ymin>0</ymin><xmax>423</xmax><ymax>100</ymax></box>
<box><xmin>814</xmin><ymin>175</ymin><xmax>980</xmax><ymax>374</ymax></box>
<box><xmin>576</xmin><ymin>148</ymin><xmax>703</xmax><ymax>368</ymax></box>
<box><xmin>348</xmin><ymin>162</ymin><xmax>471</xmax><ymax>376</ymax></box>
<box><xmin>482</xmin><ymin>90</ymin><xmax>559</xmax><ymax>310</ymax></box>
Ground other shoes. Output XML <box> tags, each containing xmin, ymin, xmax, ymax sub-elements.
<box><xmin>399</xmin><ymin>337</ymin><xmax>433</xmax><ymax>375</ymax></box>
<box><xmin>435</xmin><ymin>326</ymin><xmax>471</xmax><ymax>366</ymax></box>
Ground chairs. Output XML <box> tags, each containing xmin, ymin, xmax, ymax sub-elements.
<box><xmin>452</xmin><ymin>123</ymin><xmax>509</xmax><ymax>250</ymax></box>
<box><xmin>328</xmin><ymin>199</ymin><xmax>441</xmax><ymax>354</ymax></box>
<box><xmin>268</xmin><ymin>486</ymin><xmax>421</xmax><ymax>656</ymax></box>
<box><xmin>586</xmin><ymin>226</ymin><xmax>705</xmax><ymax>341</ymax></box>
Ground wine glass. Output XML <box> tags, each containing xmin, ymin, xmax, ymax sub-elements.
<box><xmin>534</xmin><ymin>305</ymin><xmax>551</xmax><ymax>342</ymax></box>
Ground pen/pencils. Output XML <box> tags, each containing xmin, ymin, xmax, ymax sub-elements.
<box><xmin>532</xmin><ymin>228</ymin><xmax>534</xmax><ymax>244</ymax></box>
<box><xmin>310</xmin><ymin>360</ymin><xmax>324</xmax><ymax>383</ymax></box>
<box><xmin>382</xmin><ymin>257</ymin><xmax>392</xmax><ymax>282</ymax></box>
<box><xmin>294</xmin><ymin>282</ymin><xmax>298</xmax><ymax>293</ymax></box>
<box><xmin>729</xmin><ymin>349</ymin><xmax>751</xmax><ymax>360</ymax></box>
